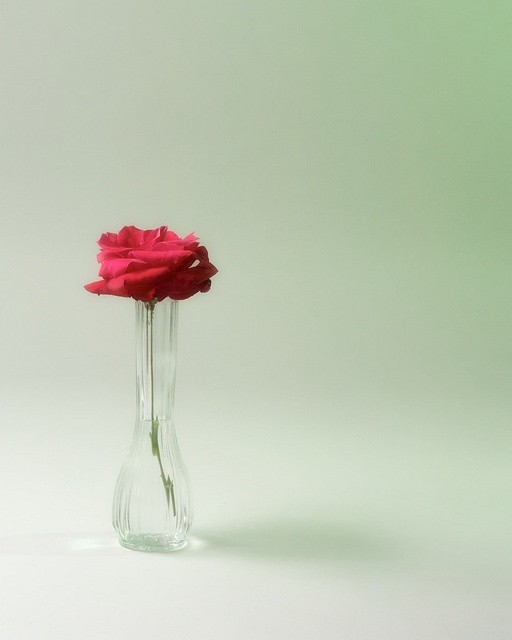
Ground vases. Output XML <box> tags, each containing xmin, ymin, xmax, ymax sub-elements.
<box><xmin>109</xmin><ymin>295</ymin><xmax>196</xmax><ymax>553</ymax></box>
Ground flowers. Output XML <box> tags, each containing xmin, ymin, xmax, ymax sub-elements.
<box><xmin>84</xmin><ymin>224</ymin><xmax>219</xmax><ymax>303</ymax></box>
